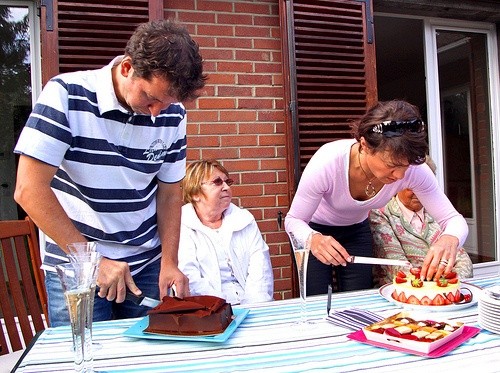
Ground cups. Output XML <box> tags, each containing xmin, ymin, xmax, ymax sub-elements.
<box><xmin>66</xmin><ymin>241</ymin><xmax>98</xmax><ymax>253</ymax></box>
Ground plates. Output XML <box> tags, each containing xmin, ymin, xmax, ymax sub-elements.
<box><xmin>121</xmin><ymin>308</ymin><xmax>251</xmax><ymax>343</ymax></box>
<box><xmin>346</xmin><ymin>319</ymin><xmax>482</xmax><ymax>359</ymax></box>
<box><xmin>477</xmin><ymin>285</ymin><xmax>500</xmax><ymax>336</ymax></box>
<box><xmin>378</xmin><ymin>281</ymin><xmax>482</xmax><ymax>312</ymax></box>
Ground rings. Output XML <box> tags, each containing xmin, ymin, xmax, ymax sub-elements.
<box><xmin>440</xmin><ymin>259</ymin><xmax>448</xmax><ymax>266</ymax></box>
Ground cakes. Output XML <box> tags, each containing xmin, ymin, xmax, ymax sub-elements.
<box><xmin>145</xmin><ymin>295</ymin><xmax>234</xmax><ymax>334</ymax></box>
<box><xmin>390</xmin><ymin>266</ymin><xmax>461</xmax><ymax>306</ymax></box>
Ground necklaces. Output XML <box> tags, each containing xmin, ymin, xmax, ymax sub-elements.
<box><xmin>358</xmin><ymin>148</ymin><xmax>376</xmax><ymax>197</ymax></box>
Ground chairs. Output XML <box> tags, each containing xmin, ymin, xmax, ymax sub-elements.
<box><xmin>0</xmin><ymin>216</ymin><xmax>49</xmax><ymax>357</ymax></box>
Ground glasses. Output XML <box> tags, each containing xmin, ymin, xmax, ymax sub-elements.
<box><xmin>366</xmin><ymin>120</ymin><xmax>424</xmax><ymax>138</ymax></box>
<box><xmin>201</xmin><ymin>177</ymin><xmax>233</xmax><ymax>186</ymax></box>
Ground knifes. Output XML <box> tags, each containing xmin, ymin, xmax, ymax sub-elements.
<box><xmin>347</xmin><ymin>256</ymin><xmax>410</xmax><ymax>267</ymax></box>
<box><xmin>125</xmin><ymin>292</ymin><xmax>162</xmax><ymax>307</ymax></box>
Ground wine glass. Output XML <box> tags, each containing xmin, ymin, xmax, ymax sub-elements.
<box><xmin>55</xmin><ymin>262</ymin><xmax>106</xmax><ymax>373</ymax></box>
<box><xmin>66</xmin><ymin>252</ymin><xmax>103</xmax><ymax>353</ymax></box>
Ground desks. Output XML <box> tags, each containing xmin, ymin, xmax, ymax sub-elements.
<box><xmin>11</xmin><ymin>272</ymin><xmax>500</xmax><ymax>373</ymax></box>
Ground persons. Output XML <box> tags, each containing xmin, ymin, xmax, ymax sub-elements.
<box><xmin>13</xmin><ymin>17</ymin><xmax>210</xmax><ymax>328</ymax></box>
<box><xmin>369</xmin><ymin>155</ymin><xmax>474</xmax><ymax>289</ymax></box>
<box><xmin>171</xmin><ymin>159</ymin><xmax>275</xmax><ymax>306</ymax></box>
<box><xmin>283</xmin><ymin>100</ymin><xmax>469</xmax><ymax>296</ymax></box>
